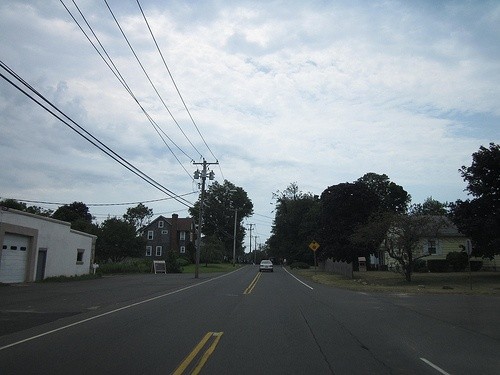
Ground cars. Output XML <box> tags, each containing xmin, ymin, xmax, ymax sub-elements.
<box><xmin>259</xmin><ymin>260</ymin><xmax>274</xmax><ymax>272</ymax></box>
